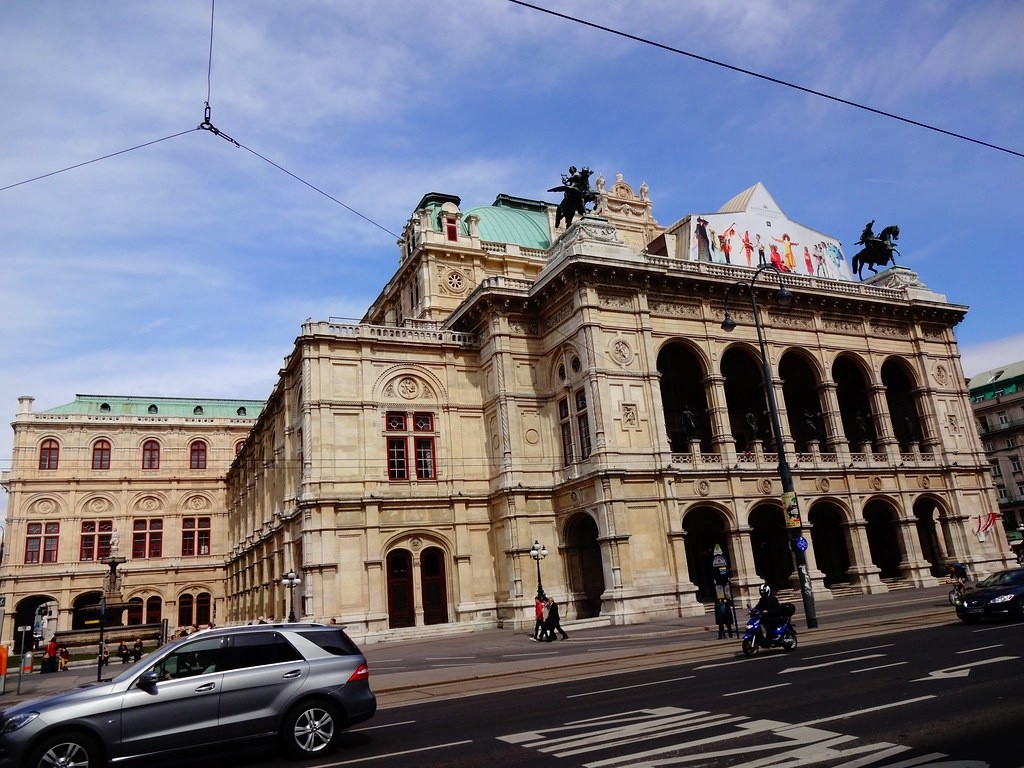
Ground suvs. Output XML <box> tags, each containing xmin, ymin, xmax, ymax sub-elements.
<box><xmin>0</xmin><ymin>621</ymin><xmax>377</xmax><ymax>768</ymax></box>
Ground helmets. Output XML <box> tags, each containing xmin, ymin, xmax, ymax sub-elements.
<box><xmin>759</xmin><ymin>584</ymin><xmax>771</xmax><ymax>598</ymax></box>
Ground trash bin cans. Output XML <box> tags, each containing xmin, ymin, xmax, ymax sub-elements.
<box><xmin>23</xmin><ymin>651</ymin><xmax>33</xmax><ymax>674</ymax></box>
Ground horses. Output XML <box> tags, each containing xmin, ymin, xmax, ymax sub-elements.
<box><xmin>851</xmin><ymin>225</ymin><xmax>901</xmax><ymax>282</ymax></box>
<box><xmin>546</xmin><ymin>166</ymin><xmax>600</xmax><ymax>230</ymax></box>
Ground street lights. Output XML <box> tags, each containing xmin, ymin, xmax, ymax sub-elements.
<box><xmin>529</xmin><ymin>540</ymin><xmax>548</xmax><ymax>602</ymax></box>
<box><xmin>721</xmin><ymin>266</ymin><xmax>819</xmax><ymax>628</ymax></box>
<box><xmin>282</xmin><ymin>568</ymin><xmax>301</xmax><ymax>622</ymax></box>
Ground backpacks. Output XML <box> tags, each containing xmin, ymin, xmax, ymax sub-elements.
<box><xmin>953</xmin><ymin>562</ymin><xmax>967</xmax><ymax>571</ymax></box>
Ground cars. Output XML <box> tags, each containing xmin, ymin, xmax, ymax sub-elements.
<box><xmin>955</xmin><ymin>567</ymin><xmax>1024</xmax><ymax>626</ymax></box>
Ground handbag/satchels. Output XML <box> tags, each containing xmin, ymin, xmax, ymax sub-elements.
<box><xmin>43</xmin><ymin>652</ymin><xmax>49</xmax><ymax>659</ymax></box>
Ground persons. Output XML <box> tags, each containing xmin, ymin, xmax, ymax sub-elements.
<box><xmin>42</xmin><ymin>637</ymin><xmax>69</xmax><ymax>673</ymax></box>
<box><xmin>258</xmin><ymin>615</ymin><xmax>274</xmax><ymax>624</ymax></box>
<box><xmin>110</xmin><ymin>528</ymin><xmax>119</xmax><ymax>546</ymax></box>
<box><xmin>104</xmin><ymin>647</ymin><xmax>109</xmax><ymax>666</ymax></box>
<box><xmin>561</xmin><ymin>165</ymin><xmax>583</xmax><ymax>210</ymax></box>
<box><xmin>753</xmin><ymin>584</ymin><xmax>782</xmax><ymax>647</ymax></box>
<box><xmin>330</xmin><ymin>619</ymin><xmax>337</xmax><ymax>624</ymax></box>
<box><xmin>165</xmin><ymin>652</ymin><xmax>217</xmax><ymax>680</ymax></box>
<box><xmin>171</xmin><ymin>622</ymin><xmax>215</xmax><ymax>672</ymax></box>
<box><xmin>118</xmin><ymin>639</ymin><xmax>143</xmax><ymax>664</ymax></box>
<box><xmin>860</xmin><ymin>220</ymin><xmax>876</xmax><ymax>261</ymax></box>
<box><xmin>715</xmin><ymin>595</ymin><xmax>734</xmax><ymax>639</ymax></box>
<box><xmin>946</xmin><ymin>565</ymin><xmax>968</xmax><ymax>594</ymax></box>
<box><xmin>529</xmin><ymin>597</ymin><xmax>569</xmax><ymax>641</ymax></box>
<box><xmin>34</xmin><ymin>631</ymin><xmax>40</xmax><ymax>650</ymax></box>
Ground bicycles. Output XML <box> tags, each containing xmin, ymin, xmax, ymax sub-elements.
<box><xmin>948</xmin><ymin>579</ymin><xmax>974</xmax><ymax>606</ymax></box>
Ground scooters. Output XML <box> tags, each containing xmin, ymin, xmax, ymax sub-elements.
<box><xmin>742</xmin><ymin>603</ymin><xmax>798</xmax><ymax>661</ymax></box>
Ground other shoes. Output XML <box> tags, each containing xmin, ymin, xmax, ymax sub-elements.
<box><xmin>552</xmin><ymin>637</ymin><xmax>557</xmax><ymax>640</ymax></box>
<box><xmin>561</xmin><ymin>636</ymin><xmax>569</xmax><ymax>640</ymax></box>
<box><xmin>542</xmin><ymin>638</ymin><xmax>547</xmax><ymax>641</ymax></box>
<box><xmin>537</xmin><ymin>638</ymin><xmax>543</xmax><ymax>642</ymax></box>
<box><xmin>529</xmin><ymin>637</ymin><xmax>536</xmax><ymax>642</ymax></box>
<box><xmin>545</xmin><ymin>639</ymin><xmax>552</xmax><ymax>642</ymax></box>
